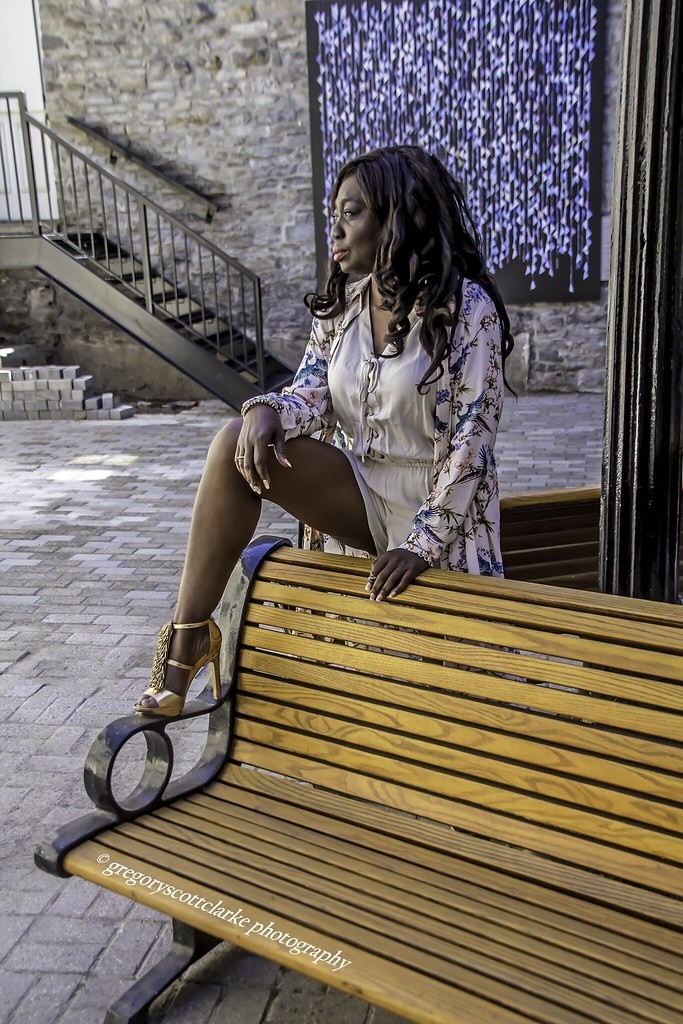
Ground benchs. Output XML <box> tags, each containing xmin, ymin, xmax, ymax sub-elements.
<box><xmin>248</xmin><ymin>461</ymin><xmax>608</xmax><ymax>606</ymax></box>
<box><xmin>34</xmin><ymin>530</ymin><xmax>683</xmax><ymax>1024</ymax></box>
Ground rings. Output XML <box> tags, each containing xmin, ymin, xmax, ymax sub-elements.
<box><xmin>237</xmin><ymin>456</ymin><xmax>245</xmax><ymax>460</ymax></box>
<box><xmin>367</xmin><ymin>573</ymin><xmax>376</xmax><ymax>584</ymax></box>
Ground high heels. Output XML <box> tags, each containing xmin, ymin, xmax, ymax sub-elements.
<box><xmin>133</xmin><ymin>614</ymin><xmax>222</xmax><ymax>717</ymax></box>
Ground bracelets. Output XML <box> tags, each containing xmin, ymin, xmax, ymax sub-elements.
<box><xmin>418</xmin><ymin>551</ymin><xmax>434</xmax><ymax>568</ymax></box>
<box><xmin>243</xmin><ymin>395</ymin><xmax>280</xmax><ymax>417</ymax></box>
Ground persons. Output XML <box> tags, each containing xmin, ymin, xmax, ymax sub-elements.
<box><xmin>134</xmin><ymin>145</ymin><xmax>519</xmax><ymax>715</ymax></box>
